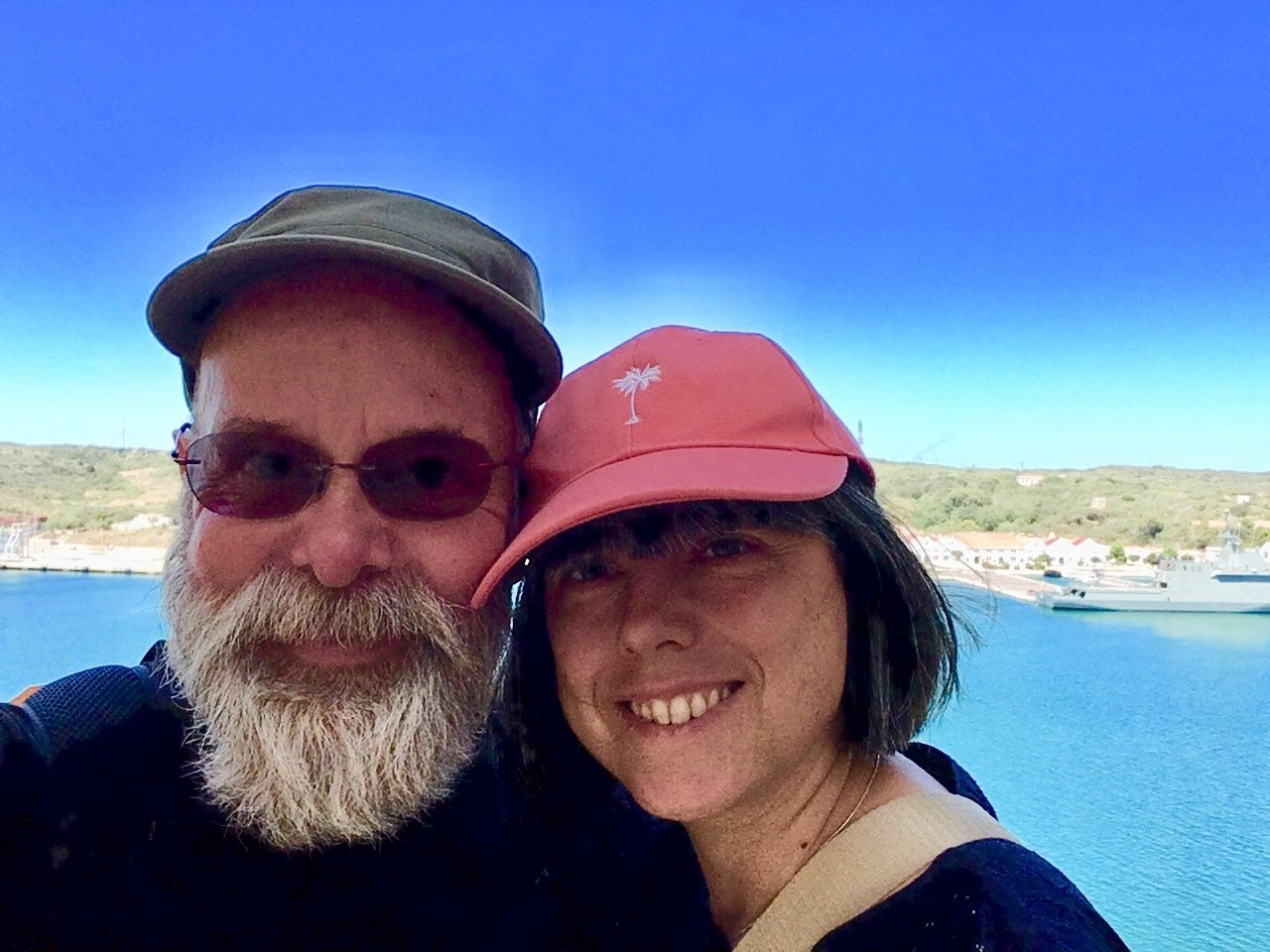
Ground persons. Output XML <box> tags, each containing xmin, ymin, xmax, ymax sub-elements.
<box><xmin>0</xmin><ymin>185</ymin><xmax>654</xmax><ymax>952</ymax></box>
<box><xmin>11</xmin><ymin>325</ymin><xmax>1129</xmax><ymax>952</ymax></box>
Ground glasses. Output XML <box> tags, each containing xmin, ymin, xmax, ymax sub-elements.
<box><xmin>170</xmin><ymin>422</ymin><xmax>521</xmax><ymax>520</ymax></box>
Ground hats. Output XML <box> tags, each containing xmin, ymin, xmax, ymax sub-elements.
<box><xmin>465</xmin><ymin>321</ymin><xmax>877</xmax><ymax>614</ymax></box>
<box><xmin>146</xmin><ymin>180</ymin><xmax>567</xmax><ymax>415</ymax></box>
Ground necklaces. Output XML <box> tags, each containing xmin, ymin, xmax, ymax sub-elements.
<box><xmin>735</xmin><ymin>752</ymin><xmax>879</xmax><ymax>938</ymax></box>
